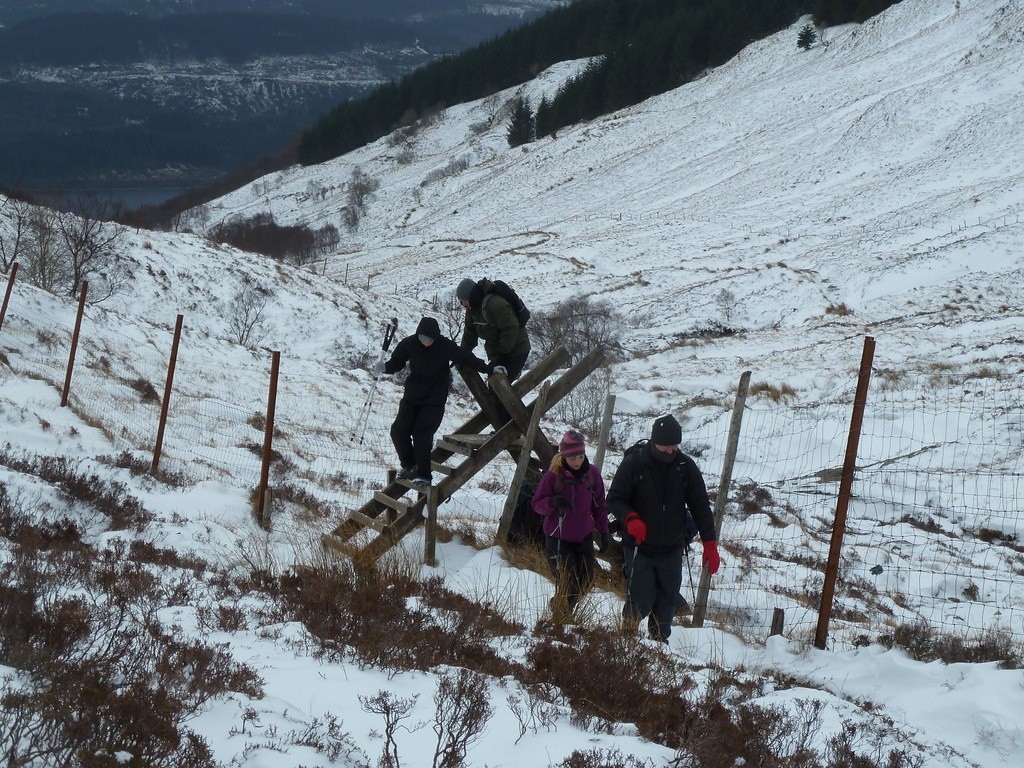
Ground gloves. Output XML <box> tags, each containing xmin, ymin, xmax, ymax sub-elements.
<box><xmin>702</xmin><ymin>540</ymin><xmax>720</xmax><ymax>574</ymax></box>
<box><xmin>624</xmin><ymin>512</ymin><xmax>647</xmax><ymax>544</ymax></box>
<box><xmin>599</xmin><ymin>533</ymin><xmax>609</xmax><ymax>553</ymax></box>
<box><xmin>551</xmin><ymin>496</ymin><xmax>571</xmax><ymax>509</ymax></box>
<box><xmin>493</xmin><ymin>365</ymin><xmax>508</xmax><ymax>376</ymax></box>
<box><xmin>376</xmin><ymin>361</ymin><xmax>386</xmax><ymax>373</ymax></box>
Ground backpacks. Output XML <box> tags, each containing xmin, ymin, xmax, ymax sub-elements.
<box><xmin>473</xmin><ymin>280</ymin><xmax>531</xmax><ymax>328</ymax></box>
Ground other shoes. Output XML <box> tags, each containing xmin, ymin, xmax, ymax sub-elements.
<box><xmin>412</xmin><ymin>478</ymin><xmax>431</xmax><ymax>487</ymax></box>
<box><xmin>396</xmin><ymin>466</ymin><xmax>416</xmax><ymax>479</ymax></box>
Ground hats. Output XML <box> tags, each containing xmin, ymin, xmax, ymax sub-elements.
<box><xmin>652</xmin><ymin>414</ymin><xmax>682</xmax><ymax>445</ymax></box>
<box><xmin>456</xmin><ymin>278</ymin><xmax>477</xmax><ymax>300</ymax></box>
<box><xmin>559</xmin><ymin>430</ymin><xmax>585</xmax><ymax>458</ymax></box>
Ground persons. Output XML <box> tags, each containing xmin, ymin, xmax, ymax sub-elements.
<box><xmin>606</xmin><ymin>414</ymin><xmax>720</xmax><ymax>647</ymax></box>
<box><xmin>375</xmin><ymin>317</ymin><xmax>508</xmax><ymax>486</ymax></box>
<box><xmin>450</xmin><ymin>278</ymin><xmax>532</xmax><ymax>440</ymax></box>
<box><xmin>531</xmin><ymin>429</ymin><xmax>607</xmax><ymax>625</ymax></box>
